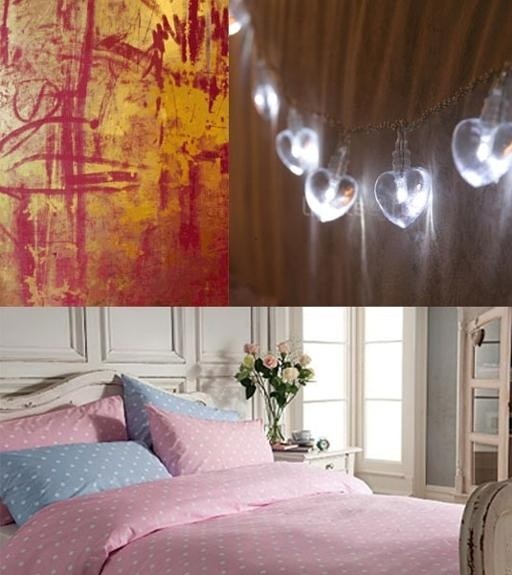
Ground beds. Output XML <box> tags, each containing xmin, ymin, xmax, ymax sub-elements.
<box><xmin>0</xmin><ymin>370</ymin><xmax>512</xmax><ymax>575</ymax></box>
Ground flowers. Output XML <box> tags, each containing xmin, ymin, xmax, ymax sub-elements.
<box><xmin>234</xmin><ymin>341</ymin><xmax>314</xmax><ymax>440</ymax></box>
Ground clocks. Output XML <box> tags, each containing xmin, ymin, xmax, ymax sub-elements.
<box><xmin>317</xmin><ymin>439</ymin><xmax>329</xmax><ymax>451</ymax></box>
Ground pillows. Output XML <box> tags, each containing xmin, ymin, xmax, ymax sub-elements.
<box><xmin>0</xmin><ymin>394</ymin><xmax>126</xmax><ymax>526</ymax></box>
<box><xmin>143</xmin><ymin>405</ymin><xmax>273</xmax><ymax>477</ymax></box>
<box><xmin>0</xmin><ymin>441</ymin><xmax>171</xmax><ymax>528</ymax></box>
<box><xmin>121</xmin><ymin>374</ymin><xmax>241</xmax><ymax>452</ymax></box>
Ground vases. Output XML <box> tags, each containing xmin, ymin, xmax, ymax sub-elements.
<box><xmin>264</xmin><ymin>397</ymin><xmax>285</xmax><ymax>444</ymax></box>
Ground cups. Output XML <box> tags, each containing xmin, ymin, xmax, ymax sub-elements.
<box><xmin>292</xmin><ymin>430</ymin><xmax>312</xmax><ymax>442</ymax></box>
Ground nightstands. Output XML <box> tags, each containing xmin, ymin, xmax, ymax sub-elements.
<box><xmin>274</xmin><ymin>447</ymin><xmax>363</xmax><ymax>476</ymax></box>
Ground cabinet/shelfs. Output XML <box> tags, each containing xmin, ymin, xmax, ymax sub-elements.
<box><xmin>454</xmin><ymin>306</ymin><xmax>512</xmax><ymax>497</ymax></box>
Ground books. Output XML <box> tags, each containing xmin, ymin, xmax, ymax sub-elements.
<box><xmin>273</xmin><ymin>439</ymin><xmax>313</xmax><ymax>452</ymax></box>
